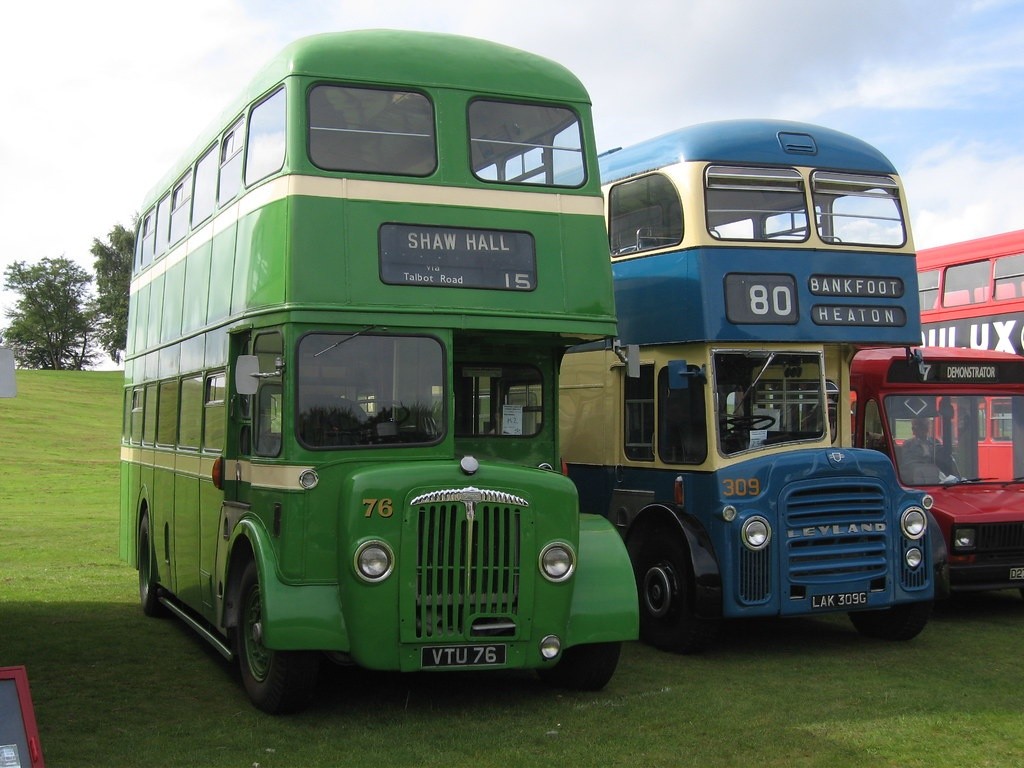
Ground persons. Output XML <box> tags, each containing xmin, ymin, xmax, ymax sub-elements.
<box><xmin>900</xmin><ymin>417</ymin><xmax>961</xmax><ymax>483</ymax></box>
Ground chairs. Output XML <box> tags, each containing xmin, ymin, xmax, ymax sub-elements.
<box><xmin>638</xmin><ymin>226</ymin><xmax>683</xmax><ymax>249</ymax></box>
<box><xmin>299</xmin><ymin>396</ymin><xmax>371</xmax><ymax>446</ymax></box>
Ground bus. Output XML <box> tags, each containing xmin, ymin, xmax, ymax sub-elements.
<box><xmin>116</xmin><ymin>29</ymin><xmax>640</xmax><ymax>712</ymax></box>
<box><xmin>750</xmin><ymin>347</ymin><xmax>1024</xmax><ymax>619</ymax></box>
<box><xmin>916</xmin><ymin>227</ymin><xmax>1024</xmax><ymax>481</ymax></box>
<box><xmin>369</xmin><ymin>118</ymin><xmax>936</xmax><ymax>642</ymax></box>
